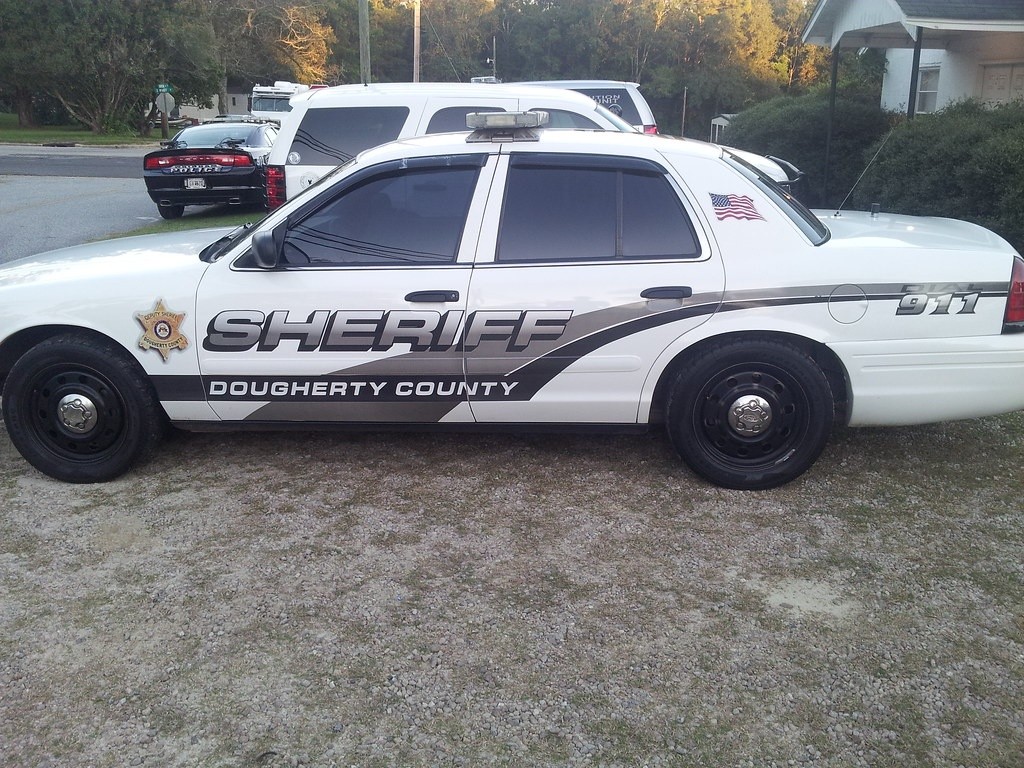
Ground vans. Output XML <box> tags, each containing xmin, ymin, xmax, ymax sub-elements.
<box><xmin>248</xmin><ymin>81</ymin><xmax>660</xmax><ymax>213</ymax></box>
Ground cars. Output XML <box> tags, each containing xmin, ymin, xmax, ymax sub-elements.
<box><xmin>143</xmin><ymin>116</ymin><xmax>281</xmax><ymax>218</ymax></box>
<box><xmin>0</xmin><ymin>111</ymin><xmax>1024</xmax><ymax>490</ymax></box>
